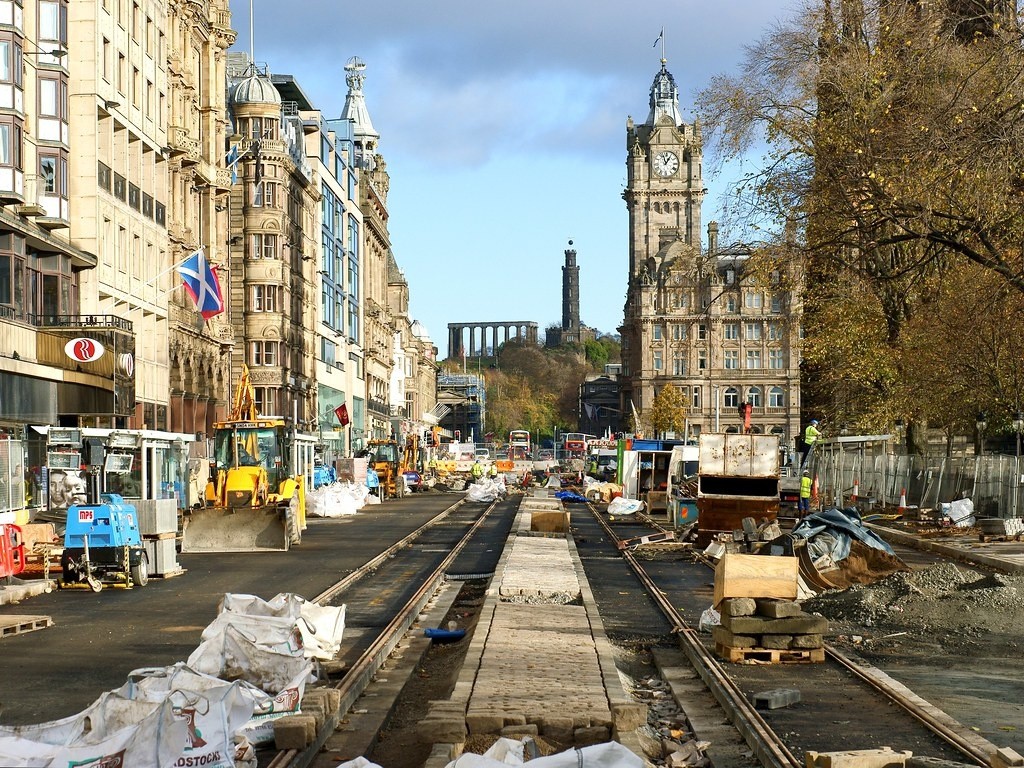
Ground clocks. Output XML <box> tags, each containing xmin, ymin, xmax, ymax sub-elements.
<box><xmin>654</xmin><ymin>151</ymin><xmax>678</xmax><ymax>176</ymax></box>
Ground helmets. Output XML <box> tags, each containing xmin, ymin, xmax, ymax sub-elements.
<box><xmin>803</xmin><ymin>470</ymin><xmax>809</xmax><ymax>474</ymax></box>
<box><xmin>492</xmin><ymin>461</ymin><xmax>496</xmax><ymax>464</ymax></box>
<box><xmin>591</xmin><ymin>457</ymin><xmax>595</xmax><ymax>461</ymax></box>
<box><xmin>811</xmin><ymin>419</ymin><xmax>818</xmax><ymax>425</ymax></box>
<box><xmin>476</xmin><ymin>460</ymin><xmax>480</xmax><ymax>463</ymax></box>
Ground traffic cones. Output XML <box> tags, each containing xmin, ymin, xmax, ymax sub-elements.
<box><xmin>898</xmin><ymin>489</ymin><xmax>909</xmax><ymax>517</ymax></box>
<box><xmin>851</xmin><ymin>478</ymin><xmax>861</xmax><ymax>502</ymax></box>
<box><xmin>578</xmin><ymin>469</ymin><xmax>584</xmax><ymax>484</ymax></box>
<box><xmin>546</xmin><ymin>464</ymin><xmax>550</xmax><ymax>478</ymax></box>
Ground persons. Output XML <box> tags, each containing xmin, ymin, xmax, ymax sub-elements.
<box><xmin>0</xmin><ymin>463</ymin><xmax>47</xmax><ymax>510</ymax></box>
<box><xmin>798</xmin><ymin>470</ymin><xmax>813</xmax><ymax>516</ymax></box>
<box><xmin>471</xmin><ymin>460</ymin><xmax>484</xmax><ymax>484</ymax></box>
<box><xmin>49</xmin><ymin>471</ymin><xmax>86</xmax><ymax>511</ymax></box>
<box><xmin>118</xmin><ymin>474</ymin><xmax>137</xmax><ymax>497</ymax></box>
<box><xmin>430</xmin><ymin>458</ymin><xmax>435</xmax><ymax>476</ymax></box>
<box><xmin>587</xmin><ymin>458</ymin><xmax>596</xmax><ymax>478</ymax></box>
<box><xmin>799</xmin><ymin>420</ymin><xmax>822</xmax><ymax>469</ymax></box>
<box><xmin>490</xmin><ymin>461</ymin><xmax>497</xmax><ymax>478</ymax></box>
<box><xmin>189</xmin><ymin>468</ymin><xmax>197</xmax><ymax>482</ymax></box>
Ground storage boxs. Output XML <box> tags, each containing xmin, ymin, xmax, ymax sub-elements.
<box><xmin>714</xmin><ymin>553</ymin><xmax>800</xmax><ymax>611</ymax></box>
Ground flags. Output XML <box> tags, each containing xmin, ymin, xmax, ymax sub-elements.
<box><xmin>181</xmin><ymin>267</ymin><xmax>224</xmax><ymax>320</ymax></box>
<box><xmin>228</xmin><ymin>132</ymin><xmax>262</xmax><ymax>187</ymax></box>
<box><xmin>335</xmin><ymin>404</ymin><xmax>350</xmax><ymax>427</ymax></box>
<box><xmin>175</xmin><ymin>250</ymin><xmax>220</xmax><ymax>313</ymax></box>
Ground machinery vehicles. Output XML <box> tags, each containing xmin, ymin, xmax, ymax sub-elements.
<box><xmin>359</xmin><ymin>433</ymin><xmax>426</xmax><ymax>500</ymax></box>
<box><xmin>181</xmin><ymin>363</ymin><xmax>310</xmax><ymax>556</ymax></box>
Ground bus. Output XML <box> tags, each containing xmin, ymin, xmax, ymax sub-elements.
<box><xmin>566</xmin><ymin>433</ymin><xmax>597</xmax><ymax>459</ymax></box>
<box><xmin>507</xmin><ymin>430</ymin><xmax>532</xmax><ymax>459</ymax></box>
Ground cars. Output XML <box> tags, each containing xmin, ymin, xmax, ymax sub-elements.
<box><xmin>476</xmin><ymin>448</ymin><xmax>491</xmax><ymax>461</ymax></box>
<box><xmin>537</xmin><ymin>451</ymin><xmax>552</xmax><ymax>461</ymax></box>
